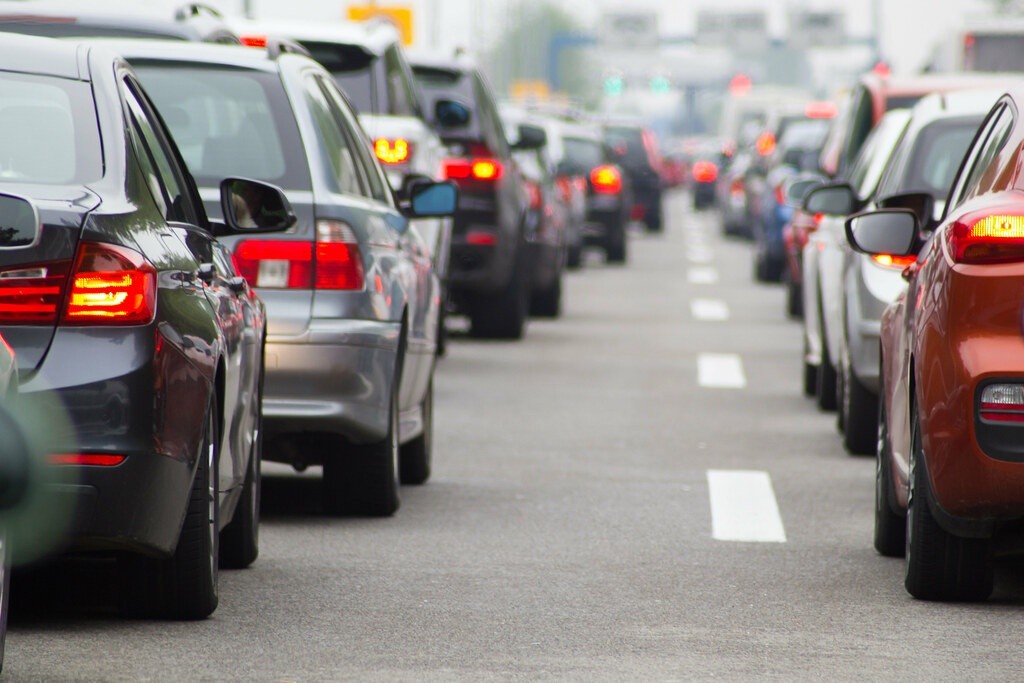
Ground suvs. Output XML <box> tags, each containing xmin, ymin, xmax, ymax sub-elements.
<box><xmin>0</xmin><ymin>0</ymin><xmax>458</xmax><ymax>519</ymax></box>
<box><xmin>406</xmin><ymin>57</ymin><xmax>547</xmax><ymax>344</ymax></box>
<box><xmin>172</xmin><ymin>1</ymin><xmax>476</xmax><ymax>488</ymax></box>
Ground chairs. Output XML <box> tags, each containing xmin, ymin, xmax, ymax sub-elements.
<box><xmin>200</xmin><ymin>115</ymin><xmax>286</xmax><ymax>180</ymax></box>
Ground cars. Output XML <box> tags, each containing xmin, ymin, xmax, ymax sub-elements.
<box><xmin>846</xmin><ymin>84</ymin><xmax>1024</xmax><ymax>602</ymax></box>
<box><xmin>801</xmin><ymin>86</ymin><xmax>1005</xmax><ymax>461</ymax></box>
<box><xmin>552</xmin><ymin>120</ymin><xmax>627</xmax><ymax>269</ymax></box>
<box><xmin>502</xmin><ymin>113</ymin><xmax>566</xmax><ymax>321</ymax></box>
<box><xmin>606</xmin><ymin>122</ymin><xmax>668</xmax><ymax>234</ymax></box>
<box><xmin>686</xmin><ymin>100</ymin><xmax>835</xmax><ymax>283</ymax></box>
<box><xmin>783</xmin><ymin>111</ymin><xmax>910</xmax><ymax>412</ymax></box>
<box><xmin>783</xmin><ymin>79</ymin><xmax>934</xmax><ymax>313</ymax></box>
<box><xmin>0</xmin><ymin>28</ymin><xmax>299</xmax><ymax>627</ymax></box>
